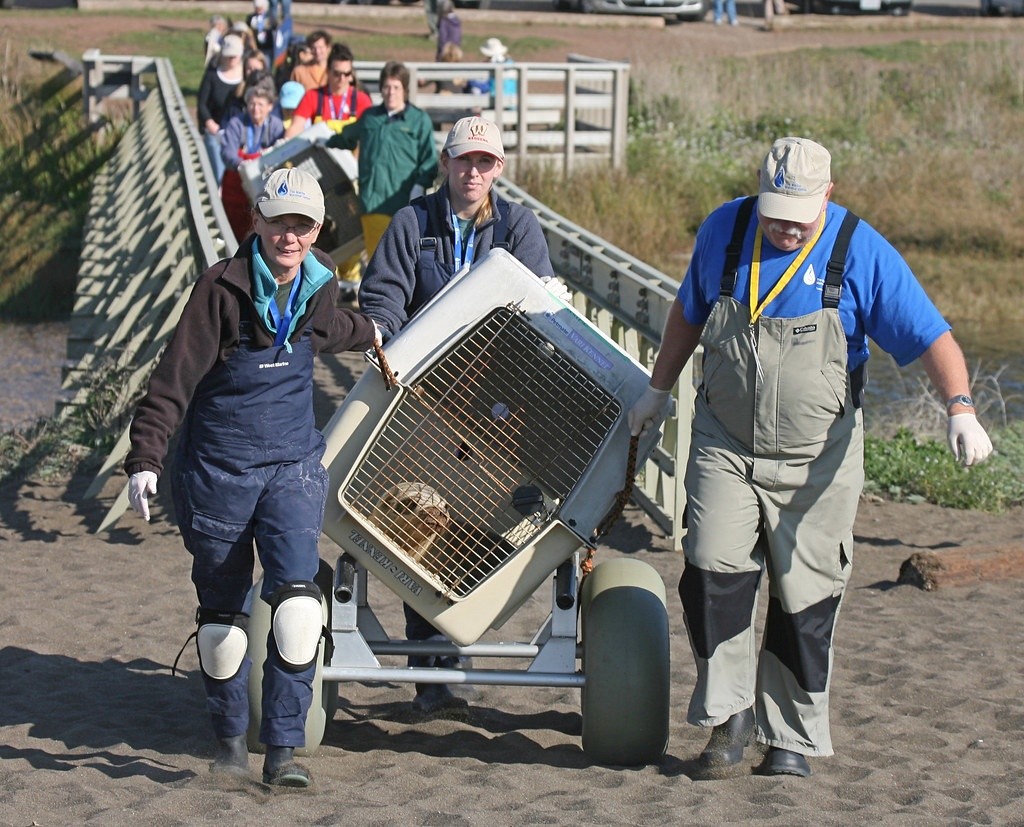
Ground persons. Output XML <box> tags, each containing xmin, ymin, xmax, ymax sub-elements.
<box><xmin>714</xmin><ymin>0</ymin><xmax>739</xmax><ymax>26</ymax></box>
<box><xmin>123</xmin><ymin>168</ymin><xmax>382</xmax><ymax>789</ymax></box>
<box><xmin>628</xmin><ymin>138</ymin><xmax>994</xmax><ymax>778</ymax></box>
<box><xmin>432</xmin><ymin>0</ymin><xmax>520</xmax><ymax>133</ymax></box>
<box><xmin>359</xmin><ymin>116</ymin><xmax>572</xmax><ymax>714</ymax></box>
<box><xmin>197</xmin><ymin>0</ymin><xmax>440</xmax><ymax>312</ymax></box>
<box><xmin>759</xmin><ymin>0</ymin><xmax>786</xmax><ymax>31</ymax></box>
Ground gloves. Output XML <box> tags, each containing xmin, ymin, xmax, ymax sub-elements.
<box><xmin>368</xmin><ymin>319</ymin><xmax>383</xmax><ymax>353</ymax></box>
<box><xmin>409</xmin><ymin>184</ymin><xmax>424</xmax><ymax>201</ymax></box>
<box><xmin>128</xmin><ymin>471</ymin><xmax>157</xmax><ymax>522</ymax></box>
<box><xmin>313</xmin><ymin>135</ymin><xmax>328</xmax><ymax>148</ymax></box>
<box><xmin>539</xmin><ymin>275</ymin><xmax>573</xmax><ymax>303</ymax></box>
<box><xmin>626</xmin><ymin>383</ymin><xmax>671</xmax><ymax>438</ymax></box>
<box><xmin>946</xmin><ymin>413</ymin><xmax>993</xmax><ymax>468</ymax></box>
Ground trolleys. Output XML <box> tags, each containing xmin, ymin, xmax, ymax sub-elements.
<box><xmin>237</xmin><ymin>121</ymin><xmax>366</xmax><ymax>311</ymax></box>
<box><xmin>246</xmin><ymin>247</ymin><xmax>678</xmax><ymax>768</ymax></box>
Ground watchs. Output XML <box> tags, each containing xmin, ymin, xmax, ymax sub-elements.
<box><xmin>946</xmin><ymin>395</ymin><xmax>976</xmax><ymax>410</ymax></box>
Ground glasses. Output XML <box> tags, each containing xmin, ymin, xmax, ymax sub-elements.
<box><xmin>331</xmin><ymin>68</ymin><xmax>352</xmax><ymax>77</ymax></box>
<box><xmin>258</xmin><ymin>210</ymin><xmax>319</xmax><ymax>239</ymax></box>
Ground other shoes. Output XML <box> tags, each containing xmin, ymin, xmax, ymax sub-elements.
<box><xmin>337</xmin><ymin>282</ymin><xmax>358</xmax><ymax>302</ymax></box>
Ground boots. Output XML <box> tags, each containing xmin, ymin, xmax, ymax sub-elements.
<box><xmin>408</xmin><ymin>636</ymin><xmax>473</xmax><ymax>720</ymax></box>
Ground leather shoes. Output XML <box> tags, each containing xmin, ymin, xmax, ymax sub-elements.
<box><xmin>696</xmin><ymin>706</ymin><xmax>758</xmax><ymax>780</ymax></box>
<box><xmin>211</xmin><ymin>731</ymin><xmax>248</xmax><ymax>778</ymax></box>
<box><xmin>262</xmin><ymin>745</ymin><xmax>308</xmax><ymax>787</ymax></box>
<box><xmin>764</xmin><ymin>745</ymin><xmax>810</xmax><ymax>777</ymax></box>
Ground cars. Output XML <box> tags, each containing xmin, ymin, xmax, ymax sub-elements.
<box><xmin>552</xmin><ymin>0</ymin><xmax>707</xmax><ymax>22</ymax></box>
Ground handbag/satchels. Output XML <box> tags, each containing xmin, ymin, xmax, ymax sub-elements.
<box><xmin>438</xmin><ymin>41</ymin><xmax>463</xmax><ymax>63</ymax></box>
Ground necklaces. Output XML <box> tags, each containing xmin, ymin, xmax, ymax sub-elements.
<box><xmin>308</xmin><ymin>64</ymin><xmax>327</xmax><ymax>88</ymax></box>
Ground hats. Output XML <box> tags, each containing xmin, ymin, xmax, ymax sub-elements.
<box><xmin>479</xmin><ymin>38</ymin><xmax>508</xmax><ymax>63</ymax></box>
<box><xmin>279</xmin><ymin>81</ymin><xmax>305</xmax><ymax>110</ymax></box>
<box><xmin>758</xmin><ymin>136</ymin><xmax>832</xmax><ymax>224</ymax></box>
<box><xmin>442</xmin><ymin>116</ymin><xmax>505</xmax><ymax>163</ymax></box>
<box><xmin>221</xmin><ymin>34</ymin><xmax>243</xmax><ymax>57</ymax></box>
<box><xmin>254</xmin><ymin>167</ymin><xmax>325</xmax><ymax>225</ymax></box>
